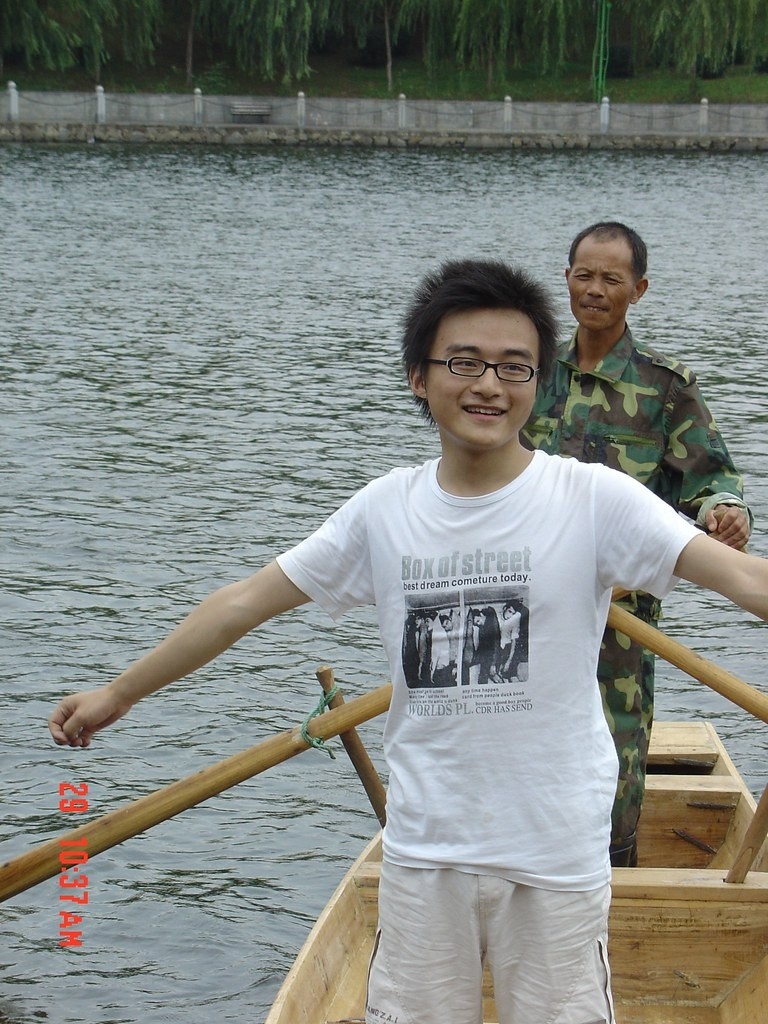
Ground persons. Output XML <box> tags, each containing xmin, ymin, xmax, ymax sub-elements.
<box><xmin>520</xmin><ymin>221</ymin><xmax>757</xmax><ymax>865</ymax></box>
<box><xmin>406</xmin><ymin>599</ymin><xmax>523</xmax><ymax>688</ymax></box>
<box><xmin>48</xmin><ymin>259</ymin><xmax>768</xmax><ymax>1023</ymax></box>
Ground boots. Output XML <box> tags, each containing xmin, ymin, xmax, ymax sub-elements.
<box><xmin>609</xmin><ymin>831</ymin><xmax>638</xmax><ymax>868</ymax></box>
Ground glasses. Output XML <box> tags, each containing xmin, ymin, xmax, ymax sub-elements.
<box><xmin>422</xmin><ymin>356</ymin><xmax>540</xmax><ymax>383</ymax></box>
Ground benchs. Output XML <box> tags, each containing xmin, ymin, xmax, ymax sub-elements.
<box><xmin>636</xmin><ymin>771</ymin><xmax>743</xmax><ymax>865</ymax></box>
